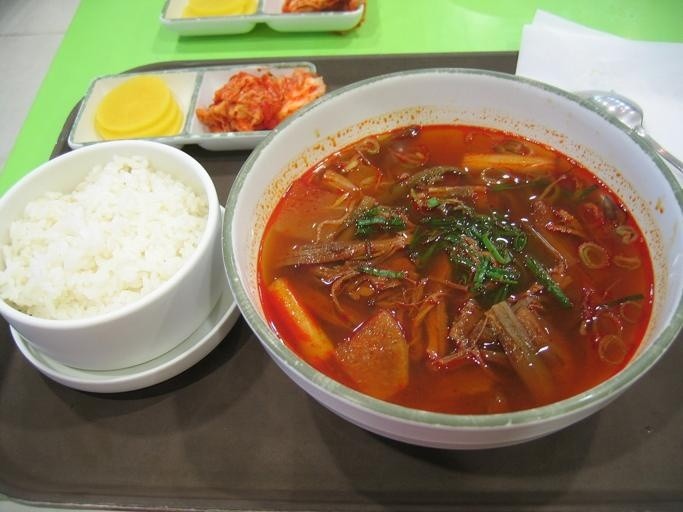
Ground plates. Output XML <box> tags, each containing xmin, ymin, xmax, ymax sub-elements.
<box><xmin>9</xmin><ymin>203</ymin><xmax>243</xmax><ymax>396</ymax></box>
<box><xmin>66</xmin><ymin>60</ymin><xmax>317</xmax><ymax>152</ymax></box>
<box><xmin>159</xmin><ymin>0</ymin><xmax>364</xmax><ymax>35</ymax></box>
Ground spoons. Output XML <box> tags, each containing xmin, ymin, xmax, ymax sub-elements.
<box><xmin>573</xmin><ymin>89</ymin><xmax>683</xmax><ymax>172</ymax></box>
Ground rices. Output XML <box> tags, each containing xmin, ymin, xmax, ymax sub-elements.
<box><xmin>0</xmin><ymin>155</ymin><xmax>209</xmax><ymax>319</ymax></box>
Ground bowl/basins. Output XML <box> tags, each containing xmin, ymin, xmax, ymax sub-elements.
<box><xmin>220</xmin><ymin>66</ymin><xmax>683</xmax><ymax>450</ymax></box>
<box><xmin>0</xmin><ymin>138</ymin><xmax>220</xmax><ymax>373</ymax></box>
